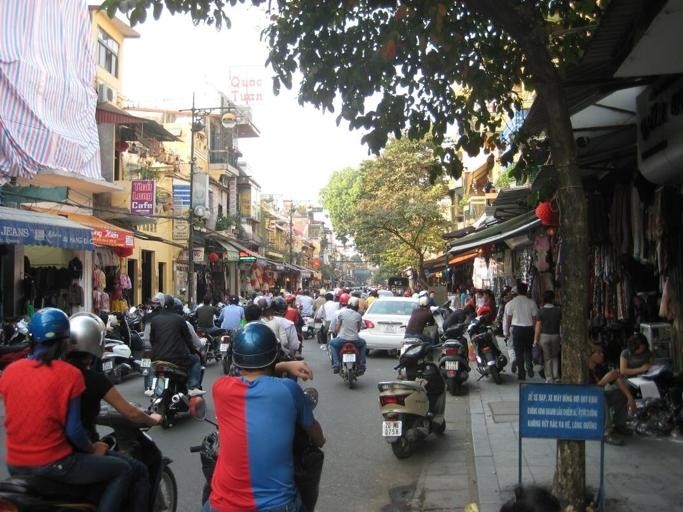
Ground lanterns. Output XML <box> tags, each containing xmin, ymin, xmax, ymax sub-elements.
<box><xmin>535</xmin><ymin>200</ymin><xmax>561</xmax><ymax>237</ymax></box>
<box><xmin>208</xmin><ymin>253</ymin><xmax>219</xmax><ymax>263</ymax></box>
<box><xmin>115</xmin><ymin>141</ymin><xmax>130</xmax><ymax>153</ymax></box>
<box><xmin>112</xmin><ymin>245</ymin><xmax>134</xmax><ymax>258</ymax></box>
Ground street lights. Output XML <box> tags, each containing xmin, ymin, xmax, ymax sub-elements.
<box><xmin>532</xmin><ymin>343</ymin><xmax>543</xmax><ymax>365</ymax></box>
<box><xmin>637</xmin><ymin>397</ymin><xmax>675</xmax><ymax>435</ymax></box>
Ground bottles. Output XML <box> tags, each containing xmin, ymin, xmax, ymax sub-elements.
<box><xmin>511</xmin><ymin>286</ymin><xmax>518</xmax><ymax>294</ymax></box>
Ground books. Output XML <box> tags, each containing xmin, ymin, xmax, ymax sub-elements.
<box><xmin>625</xmin><ymin>377</ymin><xmax>661</xmax><ymax>399</ymax></box>
<box><xmin>638</xmin><ymin>363</ymin><xmax>668</xmax><ymax>377</ymax></box>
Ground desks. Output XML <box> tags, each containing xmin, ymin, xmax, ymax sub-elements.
<box><xmin>188</xmin><ymin>107</ymin><xmax>238</xmax><ymax>305</ymax></box>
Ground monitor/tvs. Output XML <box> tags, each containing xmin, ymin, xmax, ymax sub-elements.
<box><xmin>625</xmin><ymin>377</ymin><xmax>661</xmax><ymax>399</ymax></box>
<box><xmin>638</xmin><ymin>363</ymin><xmax>668</xmax><ymax>377</ymax></box>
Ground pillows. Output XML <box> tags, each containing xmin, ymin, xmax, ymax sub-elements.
<box><xmin>231</xmin><ymin>322</ymin><xmax>278</xmax><ymax>369</ymax></box>
<box><xmin>60</xmin><ymin>312</ymin><xmax>105</xmax><ymax>361</ymax></box>
<box><xmin>253</xmin><ymin>296</ymin><xmax>271</xmax><ymax>311</ymax></box>
<box><xmin>419</xmin><ymin>297</ymin><xmax>429</xmax><ymax>306</ymax></box>
<box><xmin>28</xmin><ymin>307</ymin><xmax>69</xmax><ymax>343</ymax></box>
<box><xmin>154</xmin><ymin>293</ymin><xmax>173</xmax><ymax>310</ymax></box>
<box><xmin>284</xmin><ymin>294</ymin><xmax>293</xmax><ymax>303</ymax></box>
<box><xmin>173</xmin><ymin>298</ymin><xmax>182</xmax><ymax>314</ymax></box>
<box><xmin>347</xmin><ymin>296</ymin><xmax>358</xmax><ymax>307</ymax></box>
<box><xmin>270</xmin><ymin>297</ymin><xmax>287</xmax><ymax>315</ymax></box>
<box><xmin>339</xmin><ymin>293</ymin><xmax>350</xmax><ymax>305</ymax></box>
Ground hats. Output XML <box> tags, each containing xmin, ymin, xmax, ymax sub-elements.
<box><xmin>358</xmin><ymin>296</ymin><xmax>420</xmax><ymax>353</ymax></box>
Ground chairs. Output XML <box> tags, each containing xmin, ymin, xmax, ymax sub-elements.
<box><xmin>144</xmin><ymin>390</ymin><xmax>154</xmax><ymax>397</ymax></box>
<box><xmin>544</xmin><ymin>377</ymin><xmax>553</xmax><ymax>384</ymax></box>
<box><xmin>319</xmin><ymin>344</ymin><xmax>327</xmax><ymax>350</ymax></box>
<box><xmin>604</xmin><ymin>432</ymin><xmax>623</xmax><ymax>445</ymax></box>
<box><xmin>518</xmin><ymin>376</ymin><xmax>525</xmax><ymax>380</ymax></box>
<box><xmin>613</xmin><ymin>426</ymin><xmax>632</xmax><ymax>435</ymax></box>
<box><xmin>333</xmin><ymin>367</ymin><xmax>339</xmax><ymax>373</ymax></box>
<box><xmin>511</xmin><ymin>361</ymin><xmax>516</xmax><ymax>373</ymax></box>
<box><xmin>555</xmin><ymin>379</ymin><xmax>559</xmax><ymax>384</ymax></box>
<box><xmin>528</xmin><ymin>364</ymin><xmax>533</xmax><ymax>377</ymax></box>
<box><xmin>187</xmin><ymin>388</ymin><xmax>206</xmax><ymax>396</ymax></box>
<box><xmin>360</xmin><ymin>366</ymin><xmax>365</xmax><ymax>370</ymax></box>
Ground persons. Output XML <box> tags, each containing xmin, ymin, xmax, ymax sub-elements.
<box><xmin>587</xmin><ymin>341</ymin><xmax>637</xmax><ymax>446</ymax></box>
<box><xmin>619</xmin><ymin>333</ymin><xmax>673</xmax><ymax>400</ymax></box>
<box><xmin>0</xmin><ymin>306</ymin><xmax>135</xmax><ymax>511</ymax></box>
<box><xmin>200</xmin><ymin>323</ymin><xmax>328</xmax><ymax>512</ymax></box>
<box><xmin>0</xmin><ymin>272</ymin><xmax>564</xmax><ymax>400</ymax></box>
<box><xmin>61</xmin><ymin>310</ymin><xmax>164</xmax><ymax>510</ymax></box>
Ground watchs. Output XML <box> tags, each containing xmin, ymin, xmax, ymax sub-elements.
<box><xmin>468</xmin><ymin>312</ymin><xmax>506</xmax><ymax>384</ymax></box>
<box><xmin>141</xmin><ymin>332</ymin><xmax>206</xmax><ymax>427</ymax></box>
<box><xmin>190</xmin><ymin>387</ymin><xmax>325</xmax><ymax>511</ymax></box>
<box><xmin>439</xmin><ymin>322</ymin><xmax>469</xmax><ymax>397</ymax></box>
<box><xmin>0</xmin><ymin>382</ymin><xmax>179</xmax><ymax>512</ymax></box>
<box><xmin>376</xmin><ymin>342</ymin><xmax>447</xmax><ymax>459</ymax></box>
<box><xmin>328</xmin><ymin>340</ymin><xmax>363</xmax><ymax>390</ymax></box>
<box><xmin>98</xmin><ymin>304</ymin><xmax>142</xmax><ymax>382</ymax></box>
<box><xmin>194</xmin><ymin>316</ymin><xmax>365</xmax><ymax>373</ymax></box>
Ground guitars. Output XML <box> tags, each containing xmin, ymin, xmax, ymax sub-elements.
<box><xmin>115</xmin><ymin>141</ymin><xmax>130</xmax><ymax>153</ymax></box>
<box><xmin>112</xmin><ymin>245</ymin><xmax>134</xmax><ymax>258</ymax></box>
<box><xmin>208</xmin><ymin>253</ymin><xmax>219</xmax><ymax>263</ymax></box>
<box><xmin>535</xmin><ymin>200</ymin><xmax>561</xmax><ymax>237</ymax></box>
